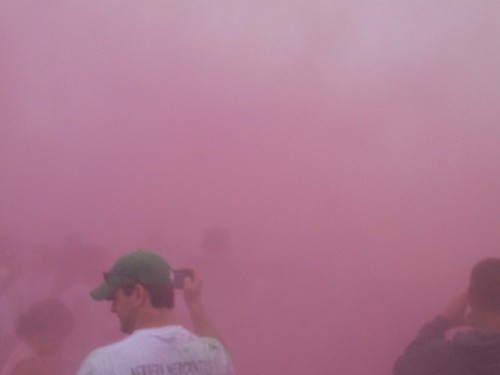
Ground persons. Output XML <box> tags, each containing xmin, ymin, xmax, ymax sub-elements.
<box><xmin>71</xmin><ymin>251</ymin><xmax>236</xmax><ymax>375</ymax></box>
<box><xmin>391</xmin><ymin>257</ymin><xmax>499</xmax><ymax>375</ymax></box>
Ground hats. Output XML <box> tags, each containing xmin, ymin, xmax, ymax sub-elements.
<box><xmin>90</xmin><ymin>250</ymin><xmax>175</xmax><ymax>301</ymax></box>
